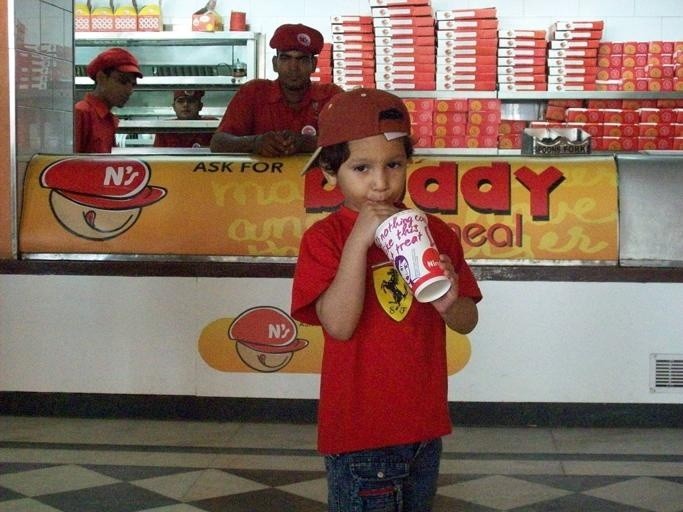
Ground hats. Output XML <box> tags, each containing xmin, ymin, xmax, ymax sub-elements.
<box><xmin>86</xmin><ymin>49</ymin><xmax>143</xmax><ymax>79</ymax></box>
<box><xmin>270</xmin><ymin>24</ymin><xmax>324</xmax><ymax>54</ymax></box>
<box><xmin>300</xmin><ymin>87</ymin><xmax>410</xmax><ymax>174</ymax></box>
<box><xmin>175</xmin><ymin>88</ymin><xmax>203</xmax><ymax>98</ymax></box>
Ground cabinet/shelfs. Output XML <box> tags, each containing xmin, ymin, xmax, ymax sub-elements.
<box><xmin>74</xmin><ymin>31</ymin><xmax>266</xmax><ymax>155</ymax></box>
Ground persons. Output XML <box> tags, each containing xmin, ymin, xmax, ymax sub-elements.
<box><xmin>74</xmin><ymin>49</ymin><xmax>143</xmax><ymax>153</ymax></box>
<box><xmin>291</xmin><ymin>89</ymin><xmax>482</xmax><ymax>512</ymax></box>
<box><xmin>154</xmin><ymin>90</ymin><xmax>218</xmax><ymax>147</ymax></box>
<box><xmin>210</xmin><ymin>23</ymin><xmax>344</xmax><ymax>155</ymax></box>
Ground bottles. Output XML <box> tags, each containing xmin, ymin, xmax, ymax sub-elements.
<box><xmin>232</xmin><ymin>68</ymin><xmax>245</xmax><ymax>77</ymax></box>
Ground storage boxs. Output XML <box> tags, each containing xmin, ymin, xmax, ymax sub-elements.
<box><xmin>310</xmin><ymin>0</ymin><xmax>682</xmax><ymax>150</ymax></box>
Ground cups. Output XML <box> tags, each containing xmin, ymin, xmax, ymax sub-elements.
<box><xmin>372</xmin><ymin>209</ymin><xmax>451</xmax><ymax>303</ymax></box>
<box><xmin>229</xmin><ymin>11</ymin><xmax>246</xmax><ymax>31</ymax></box>
